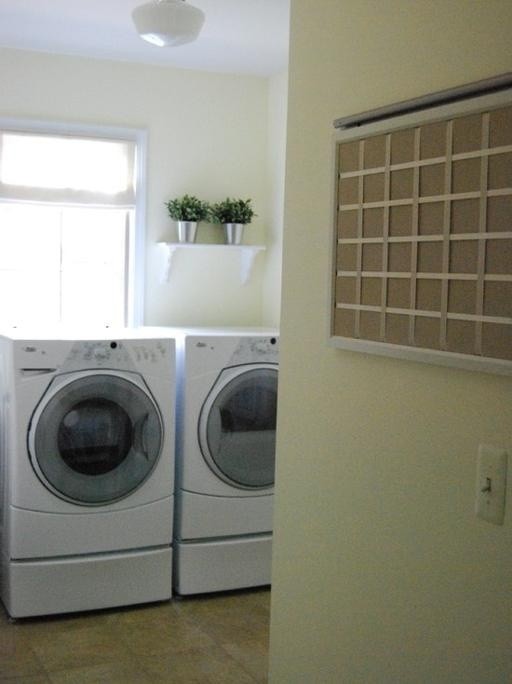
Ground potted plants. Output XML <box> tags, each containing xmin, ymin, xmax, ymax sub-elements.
<box><xmin>169</xmin><ymin>197</ymin><xmax>209</xmax><ymax>241</ymax></box>
<box><xmin>212</xmin><ymin>197</ymin><xmax>256</xmax><ymax>244</ymax></box>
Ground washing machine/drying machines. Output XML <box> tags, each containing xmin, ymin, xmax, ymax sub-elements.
<box><xmin>174</xmin><ymin>328</ymin><xmax>280</xmax><ymax>597</ymax></box>
<box><xmin>0</xmin><ymin>328</ymin><xmax>177</xmax><ymax>617</ymax></box>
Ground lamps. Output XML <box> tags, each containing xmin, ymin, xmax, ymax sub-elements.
<box><xmin>131</xmin><ymin>1</ymin><xmax>206</xmax><ymax>45</ymax></box>
